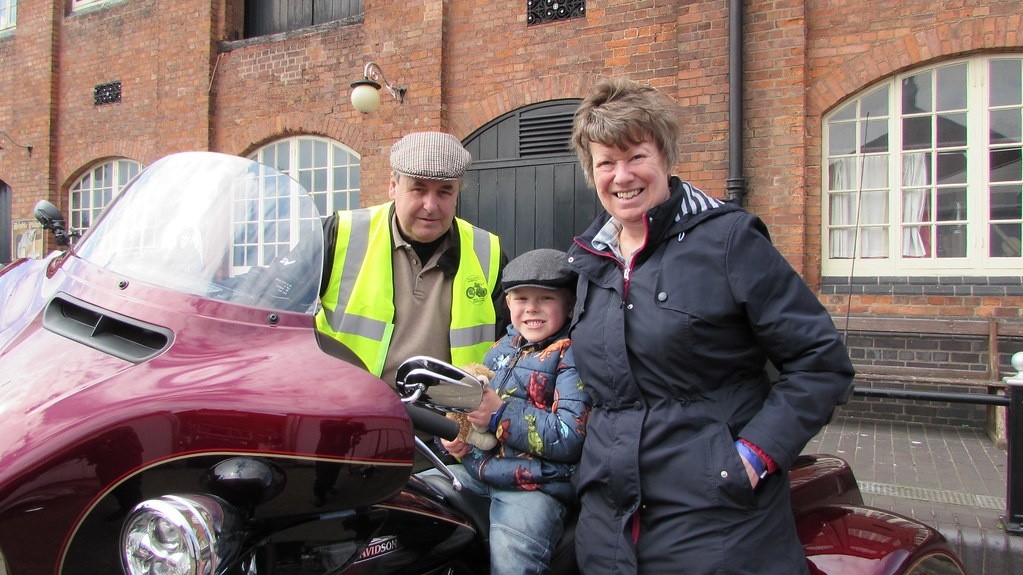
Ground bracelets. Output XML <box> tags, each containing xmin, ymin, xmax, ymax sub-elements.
<box><xmin>734</xmin><ymin>440</ymin><xmax>767</xmax><ymax>480</ymax></box>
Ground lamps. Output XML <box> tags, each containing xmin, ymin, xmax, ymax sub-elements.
<box><xmin>349</xmin><ymin>61</ymin><xmax>406</xmax><ymax>115</ymax></box>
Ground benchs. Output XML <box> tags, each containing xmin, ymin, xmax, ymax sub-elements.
<box><xmin>830</xmin><ymin>315</ymin><xmax>1022</xmax><ymax>450</ymax></box>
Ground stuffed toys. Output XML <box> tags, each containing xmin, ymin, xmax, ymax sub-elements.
<box><xmin>442</xmin><ymin>362</ymin><xmax>498</xmax><ymax>450</ymax></box>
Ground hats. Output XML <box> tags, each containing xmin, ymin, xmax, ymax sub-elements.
<box><xmin>389</xmin><ymin>132</ymin><xmax>472</xmax><ymax>181</ymax></box>
<box><xmin>501</xmin><ymin>249</ymin><xmax>575</xmax><ymax>293</ymax></box>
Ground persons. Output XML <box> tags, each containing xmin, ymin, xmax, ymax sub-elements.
<box><xmin>200</xmin><ymin>128</ymin><xmax>512</xmax><ymax>413</ymax></box>
<box><xmin>417</xmin><ymin>247</ymin><xmax>593</xmax><ymax>575</ymax></box>
<box><xmin>560</xmin><ymin>80</ymin><xmax>856</xmax><ymax>575</ymax></box>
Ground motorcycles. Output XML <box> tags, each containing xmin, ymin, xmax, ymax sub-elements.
<box><xmin>0</xmin><ymin>149</ymin><xmax>973</xmax><ymax>574</ymax></box>
